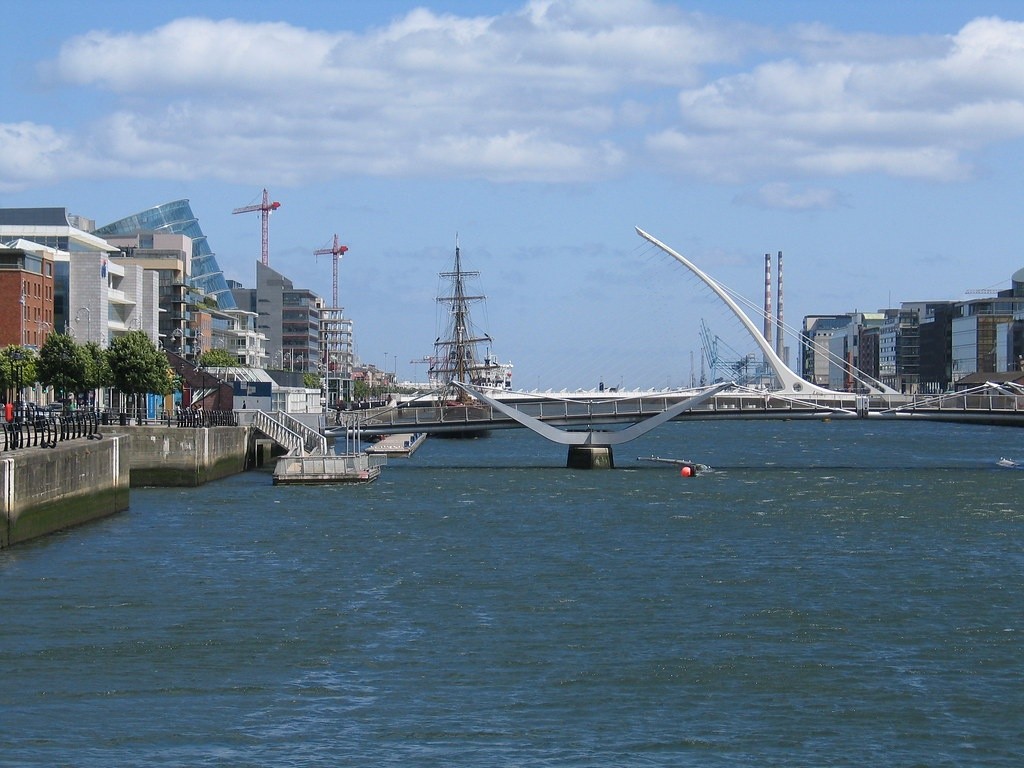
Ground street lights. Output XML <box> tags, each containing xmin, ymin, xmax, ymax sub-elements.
<box><xmin>384</xmin><ymin>352</ymin><xmax>388</xmax><ymax>371</ymax></box>
<box><xmin>394</xmin><ymin>356</ymin><xmax>397</xmax><ymax>373</ymax></box>
<box><xmin>192</xmin><ymin>333</ymin><xmax>204</xmax><ymax>353</ymax></box>
<box><xmin>172</xmin><ymin>329</ymin><xmax>183</xmax><ymax>357</ymax></box>
<box><xmin>76</xmin><ymin>306</ymin><xmax>90</xmax><ymax>342</ymax></box>
<box><xmin>129</xmin><ymin>317</ymin><xmax>142</xmax><ymax>330</ymax></box>
<box><xmin>12</xmin><ymin>349</ymin><xmax>22</xmax><ymax>431</ymax></box>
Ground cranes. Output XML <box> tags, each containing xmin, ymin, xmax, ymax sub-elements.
<box><xmin>313</xmin><ymin>235</ymin><xmax>349</xmax><ymax>307</ymax></box>
<box><xmin>232</xmin><ymin>188</ymin><xmax>282</xmax><ymax>266</ymax></box>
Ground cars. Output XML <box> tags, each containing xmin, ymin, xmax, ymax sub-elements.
<box><xmin>0</xmin><ymin>401</ymin><xmax>67</xmax><ymax>419</ymax></box>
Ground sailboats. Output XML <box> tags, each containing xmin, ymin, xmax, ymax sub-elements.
<box><xmin>428</xmin><ymin>233</ymin><xmax>500</xmax><ymax>437</ymax></box>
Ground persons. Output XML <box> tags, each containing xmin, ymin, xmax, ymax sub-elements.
<box><xmin>242</xmin><ymin>400</ymin><xmax>246</xmax><ymax>409</ymax></box>
<box><xmin>191</xmin><ymin>404</ymin><xmax>202</xmax><ymax>419</ymax></box>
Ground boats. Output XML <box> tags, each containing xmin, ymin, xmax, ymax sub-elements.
<box><xmin>997</xmin><ymin>457</ymin><xmax>1020</xmax><ymax>468</ymax></box>
